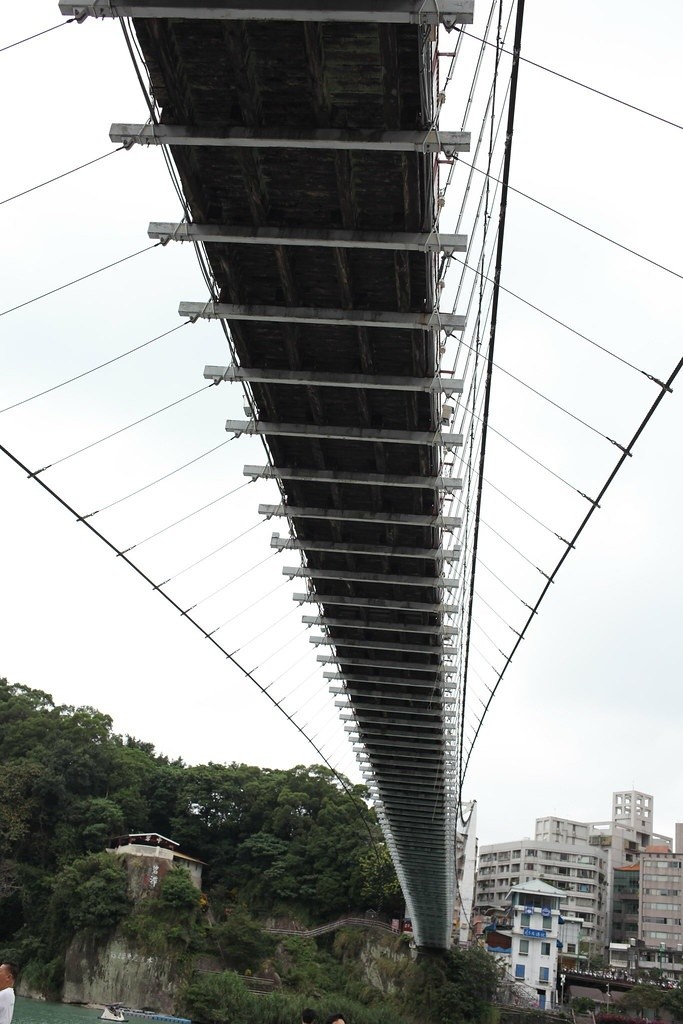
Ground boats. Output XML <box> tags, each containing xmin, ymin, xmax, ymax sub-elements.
<box><xmin>97</xmin><ymin>1002</ymin><xmax>126</xmax><ymax>1021</ymax></box>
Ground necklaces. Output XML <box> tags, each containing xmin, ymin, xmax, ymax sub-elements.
<box><xmin>0</xmin><ymin>986</ymin><xmax>10</xmax><ymax>991</ymax></box>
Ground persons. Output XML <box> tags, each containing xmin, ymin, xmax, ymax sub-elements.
<box><xmin>0</xmin><ymin>962</ymin><xmax>19</xmax><ymax>1024</ymax></box>
<box><xmin>325</xmin><ymin>1014</ymin><xmax>346</xmax><ymax>1024</ymax></box>
<box><xmin>620</xmin><ymin>969</ymin><xmax>627</xmax><ymax>980</ymax></box>
<box><xmin>301</xmin><ymin>1008</ymin><xmax>316</xmax><ymax>1024</ymax></box>
